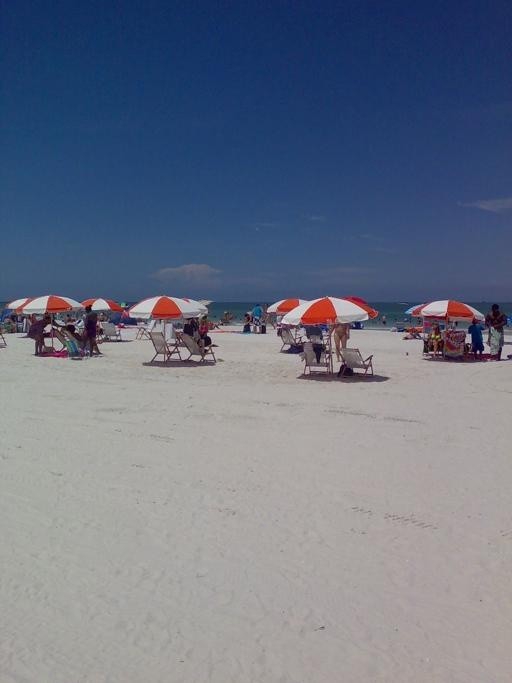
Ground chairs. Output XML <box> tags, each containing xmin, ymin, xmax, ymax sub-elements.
<box><xmin>278</xmin><ymin>323</ymin><xmax>375</xmax><ymax>378</ymax></box>
<box><xmin>137</xmin><ymin>318</ymin><xmax>218</xmax><ymax>365</ymax></box>
<box><xmin>441</xmin><ymin>329</ymin><xmax>473</xmax><ymax>359</ymax></box>
<box><xmin>52</xmin><ymin>320</ymin><xmax>122</xmax><ymax>359</ymax></box>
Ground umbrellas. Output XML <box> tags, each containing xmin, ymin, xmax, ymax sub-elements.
<box><xmin>4</xmin><ymin>294</ymin><xmax>124</xmax><ymax>352</ymax></box>
<box><xmin>128</xmin><ymin>294</ymin><xmax>209</xmax><ymax>363</ymax></box>
<box><xmin>405</xmin><ymin>298</ymin><xmax>485</xmax><ymax>361</ymax></box>
<box><xmin>266</xmin><ymin>294</ymin><xmax>378</xmax><ymax>374</ymax></box>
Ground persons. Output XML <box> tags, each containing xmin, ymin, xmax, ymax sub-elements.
<box><xmin>253</xmin><ymin>304</ymin><xmax>263</xmax><ymax>319</ymax></box>
<box><xmin>27</xmin><ymin>317</ymin><xmax>51</xmax><ymax>355</ymax></box>
<box><xmin>485</xmin><ymin>304</ymin><xmax>507</xmax><ymax>360</ymax></box>
<box><xmin>84</xmin><ymin>305</ymin><xmax>102</xmax><ymax>358</ymax></box>
<box><xmin>243</xmin><ymin>311</ymin><xmax>253</xmax><ymax>324</ymax></box>
